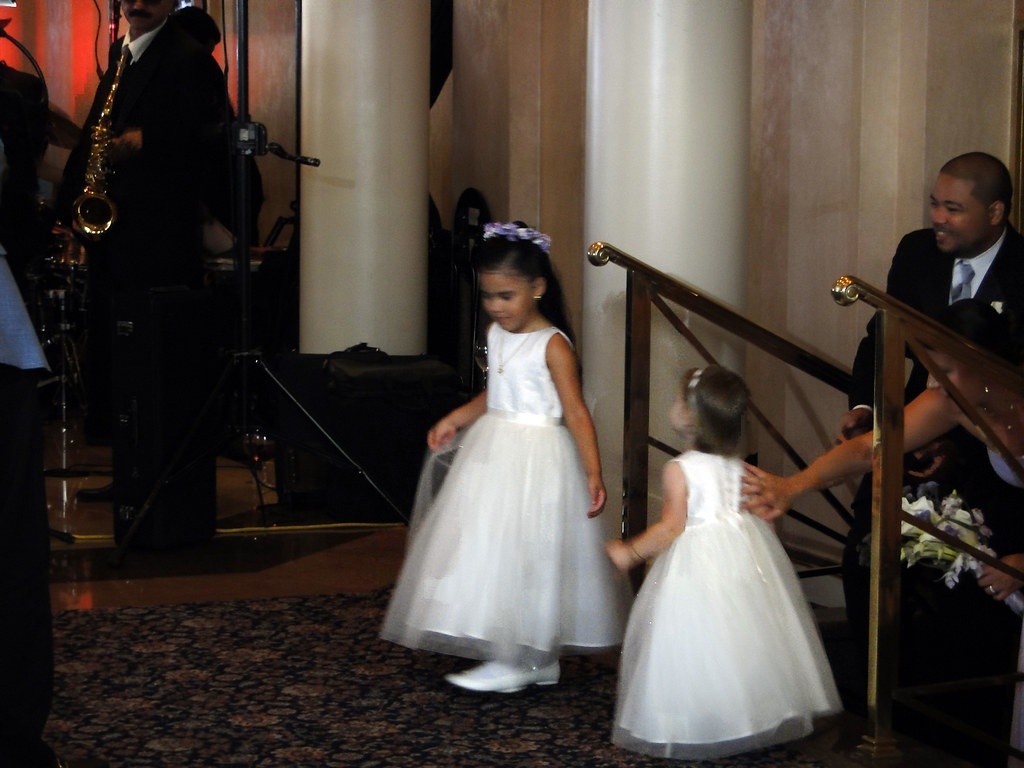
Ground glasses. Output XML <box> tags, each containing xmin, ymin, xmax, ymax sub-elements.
<box><xmin>124</xmin><ymin>0</ymin><xmax>161</xmax><ymax>6</ymax></box>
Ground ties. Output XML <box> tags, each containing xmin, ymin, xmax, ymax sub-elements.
<box><xmin>951</xmin><ymin>260</ymin><xmax>975</xmax><ymax>303</ymax></box>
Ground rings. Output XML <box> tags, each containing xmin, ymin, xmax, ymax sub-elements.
<box><xmin>989</xmin><ymin>585</ymin><xmax>995</xmax><ymax>593</ymax></box>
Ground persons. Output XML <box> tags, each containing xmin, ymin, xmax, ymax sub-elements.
<box><xmin>836</xmin><ymin>153</ymin><xmax>1024</xmax><ymax>767</ymax></box>
<box><xmin>0</xmin><ymin>69</ymin><xmax>111</xmax><ymax>767</ymax></box>
<box><xmin>605</xmin><ymin>363</ymin><xmax>842</xmax><ymax>758</ymax></box>
<box><xmin>381</xmin><ymin>218</ymin><xmax>635</xmax><ymax>692</ymax></box>
<box><xmin>743</xmin><ymin>298</ymin><xmax>1024</xmax><ymax>601</ymax></box>
<box><xmin>57</xmin><ymin>0</ymin><xmax>264</xmax><ymax>570</ymax></box>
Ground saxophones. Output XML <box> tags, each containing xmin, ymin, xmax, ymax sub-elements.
<box><xmin>72</xmin><ymin>43</ymin><xmax>130</xmax><ymax>237</ymax></box>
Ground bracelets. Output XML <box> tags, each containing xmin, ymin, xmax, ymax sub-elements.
<box><xmin>632</xmin><ymin>546</ymin><xmax>644</xmax><ymax>560</ymax></box>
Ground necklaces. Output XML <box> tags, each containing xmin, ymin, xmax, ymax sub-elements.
<box><xmin>496</xmin><ymin>324</ymin><xmax>535</xmax><ymax>374</ymax></box>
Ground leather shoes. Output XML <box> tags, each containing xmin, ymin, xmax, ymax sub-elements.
<box><xmin>445</xmin><ymin>662</ymin><xmax>560</xmax><ymax>692</ymax></box>
<box><xmin>76</xmin><ymin>482</ymin><xmax>115</xmax><ymax>502</ymax></box>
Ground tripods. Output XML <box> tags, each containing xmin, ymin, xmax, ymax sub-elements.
<box><xmin>202</xmin><ymin>0</ymin><xmax>409</xmax><ymax>530</ymax></box>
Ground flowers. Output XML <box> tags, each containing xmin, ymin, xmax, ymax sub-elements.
<box><xmin>990</xmin><ymin>301</ymin><xmax>1003</xmax><ymax>314</ymax></box>
<box><xmin>482</xmin><ymin>221</ymin><xmax>551</xmax><ymax>252</ymax></box>
<box><xmin>902</xmin><ymin>489</ymin><xmax>1024</xmax><ymax>616</ymax></box>
